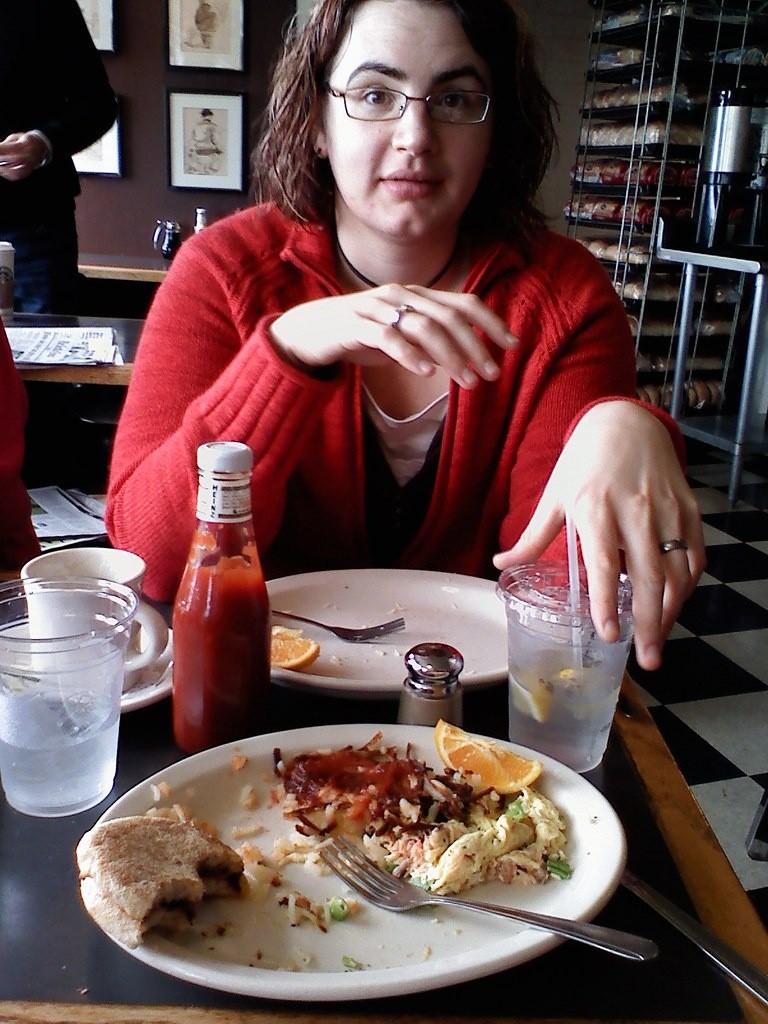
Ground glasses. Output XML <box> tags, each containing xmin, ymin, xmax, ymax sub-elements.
<box><xmin>324</xmin><ymin>82</ymin><xmax>494</xmax><ymax>124</ymax></box>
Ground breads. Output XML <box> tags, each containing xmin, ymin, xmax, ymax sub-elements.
<box><xmin>567</xmin><ymin>3</ymin><xmax>767</xmax><ymax>413</ymax></box>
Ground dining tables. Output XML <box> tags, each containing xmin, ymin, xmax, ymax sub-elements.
<box><xmin>76</xmin><ymin>252</ymin><xmax>172</xmax><ymax>282</ymax></box>
<box><xmin>1</xmin><ymin>568</ymin><xmax>767</xmax><ymax>1024</ymax></box>
<box><xmin>3</xmin><ymin>312</ymin><xmax>146</xmax><ymax>385</ymax></box>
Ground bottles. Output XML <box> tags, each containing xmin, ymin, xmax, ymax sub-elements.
<box><xmin>700</xmin><ymin>88</ymin><xmax>754</xmax><ymax>184</ymax></box>
<box><xmin>396</xmin><ymin>642</ymin><xmax>464</xmax><ymax>730</ymax></box>
<box><xmin>173</xmin><ymin>441</ymin><xmax>274</xmax><ymax>752</ymax></box>
<box><xmin>153</xmin><ymin>220</ymin><xmax>183</xmax><ymax>260</ymax></box>
<box><xmin>193</xmin><ymin>208</ymin><xmax>207</xmax><ymax>234</ymax></box>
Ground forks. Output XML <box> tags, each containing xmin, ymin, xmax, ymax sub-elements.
<box><xmin>318</xmin><ymin>835</ymin><xmax>658</xmax><ymax>961</ymax></box>
<box><xmin>272</xmin><ymin>609</ymin><xmax>404</xmax><ymax>643</ymax></box>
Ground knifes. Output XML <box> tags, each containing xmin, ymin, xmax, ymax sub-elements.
<box><xmin>619</xmin><ymin>871</ymin><xmax>768</xmax><ymax>1007</ymax></box>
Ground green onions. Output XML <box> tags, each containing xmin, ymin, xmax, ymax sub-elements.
<box><xmin>329</xmin><ymin>801</ymin><xmax>572</xmax><ymax>919</ymax></box>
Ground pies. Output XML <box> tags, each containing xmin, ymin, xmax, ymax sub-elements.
<box><xmin>76</xmin><ymin>816</ymin><xmax>253</xmax><ymax>946</ymax></box>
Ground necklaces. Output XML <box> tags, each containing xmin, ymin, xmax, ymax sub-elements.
<box><xmin>337</xmin><ymin>220</ymin><xmax>458</xmax><ymax>289</ymax></box>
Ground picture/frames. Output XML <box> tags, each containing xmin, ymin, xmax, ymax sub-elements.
<box><xmin>164</xmin><ymin>85</ymin><xmax>249</xmax><ymax>196</ymax></box>
<box><xmin>77</xmin><ymin>0</ymin><xmax>118</xmax><ymax>56</ymax></box>
<box><xmin>71</xmin><ymin>94</ymin><xmax>124</xmax><ymax>180</ymax></box>
<box><xmin>163</xmin><ymin>0</ymin><xmax>250</xmax><ymax>76</ymax></box>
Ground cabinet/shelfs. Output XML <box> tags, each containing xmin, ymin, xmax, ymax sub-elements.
<box><xmin>657</xmin><ymin>217</ymin><xmax>768</xmax><ymax>512</ymax></box>
<box><xmin>564</xmin><ymin>0</ymin><xmax>768</xmax><ymax>412</ymax></box>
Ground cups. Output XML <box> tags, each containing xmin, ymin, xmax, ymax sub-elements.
<box><xmin>496</xmin><ymin>559</ymin><xmax>634</xmax><ymax>772</ymax></box>
<box><xmin>0</xmin><ymin>577</ymin><xmax>139</xmax><ymax>818</ymax></box>
<box><xmin>21</xmin><ymin>547</ymin><xmax>168</xmax><ymax>692</ymax></box>
<box><xmin>0</xmin><ymin>241</ymin><xmax>16</xmax><ymax>321</ymax></box>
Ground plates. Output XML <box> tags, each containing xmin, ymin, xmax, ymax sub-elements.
<box><xmin>91</xmin><ymin>724</ymin><xmax>628</xmax><ymax>1001</ymax></box>
<box><xmin>265</xmin><ymin>568</ymin><xmax>509</xmax><ymax>700</ymax></box>
<box><xmin>0</xmin><ymin>624</ymin><xmax>172</xmax><ymax>712</ymax></box>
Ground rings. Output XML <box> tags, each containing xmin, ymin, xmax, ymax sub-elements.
<box><xmin>660</xmin><ymin>539</ymin><xmax>688</xmax><ymax>555</ymax></box>
<box><xmin>390</xmin><ymin>304</ymin><xmax>417</xmax><ymax>330</ymax></box>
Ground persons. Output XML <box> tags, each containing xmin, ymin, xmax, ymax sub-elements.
<box><xmin>0</xmin><ymin>0</ymin><xmax>117</xmax><ymax>311</ymax></box>
<box><xmin>105</xmin><ymin>0</ymin><xmax>705</xmax><ymax>670</ymax></box>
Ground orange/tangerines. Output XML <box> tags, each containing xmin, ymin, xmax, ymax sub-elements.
<box><xmin>270</xmin><ymin>625</ymin><xmax>320</xmax><ymax>670</ymax></box>
<box><xmin>435</xmin><ymin>720</ymin><xmax>543</xmax><ymax>794</ymax></box>
<box><xmin>507</xmin><ymin>667</ymin><xmax>552</xmax><ymax>723</ymax></box>
